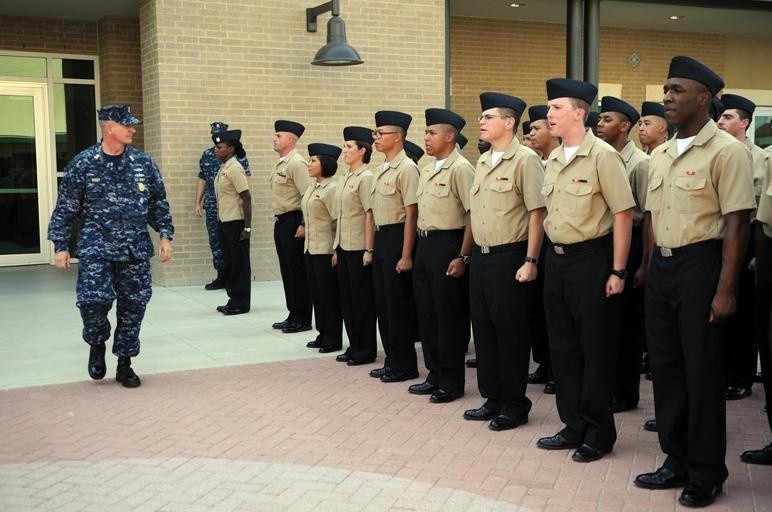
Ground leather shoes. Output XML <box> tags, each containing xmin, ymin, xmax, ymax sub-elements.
<box><xmin>306</xmin><ymin>336</ymin><xmax>323</xmax><ymax>347</ymax></box>
<box><xmin>634</xmin><ymin>464</ymin><xmax>686</xmax><ymax>490</ymax></box>
<box><xmin>645</xmin><ymin>372</ymin><xmax>653</xmax><ymax>380</ymax></box>
<box><xmin>319</xmin><ymin>342</ymin><xmax>342</xmax><ymax>353</ymax></box>
<box><xmin>368</xmin><ymin>366</ymin><xmax>392</xmax><ymax>377</ymax></box>
<box><xmin>640</xmin><ymin>359</ymin><xmax>650</xmax><ymax>373</ymax></box>
<box><xmin>429</xmin><ymin>384</ymin><xmax>464</xmax><ymax>403</ymax></box>
<box><xmin>463</xmin><ymin>404</ymin><xmax>499</xmax><ymax>421</ymax></box>
<box><xmin>644</xmin><ymin>419</ymin><xmax>657</xmax><ymax>432</ymax></box>
<box><xmin>527</xmin><ymin>373</ymin><xmax>550</xmax><ymax>384</ymax></box>
<box><xmin>336</xmin><ymin>352</ymin><xmax>353</xmax><ymax>362</ymax></box>
<box><xmin>543</xmin><ymin>379</ymin><xmax>557</xmax><ymax>394</ymax></box>
<box><xmin>572</xmin><ymin>441</ymin><xmax>613</xmax><ymax>462</ymax></box>
<box><xmin>283</xmin><ymin>319</ymin><xmax>312</xmax><ymax>333</ymax></box>
<box><xmin>726</xmin><ymin>387</ymin><xmax>753</xmax><ymax>400</ymax></box>
<box><xmin>740</xmin><ymin>442</ymin><xmax>772</xmax><ymax>466</ymax></box>
<box><xmin>217</xmin><ymin>299</ymin><xmax>250</xmax><ymax>315</ymax></box>
<box><xmin>408</xmin><ymin>381</ymin><xmax>437</xmax><ymax>394</ymax></box>
<box><xmin>379</xmin><ymin>369</ymin><xmax>419</xmax><ymax>383</ymax></box>
<box><xmin>283</xmin><ymin>309</ymin><xmax>284</xmax><ymax>316</ymax></box>
<box><xmin>466</xmin><ymin>358</ymin><xmax>476</xmax><ymax>368</ymax></box>
<box><xmin>535</xmin><ymin>433</ymin><xmax>582</xmax><ymax>449</ymax></box>
<box><xmin>678</xmin><ymin>479</ymin><xmax>722</xmax><ymax>507</ymax></box>
<box><xmin>347</xmin><ymin>356</ymin><xmax>377</xmax><ymax>366</ymax></box>
<box><xmin>488</xmin><ymin>411</ymin><xmax>529</xmax><ymax>431</ymax></box>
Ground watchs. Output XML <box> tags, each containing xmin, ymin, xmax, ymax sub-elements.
<box><xmin>243</xmin><ymin>227</ymin><xmax>252</xmax><ymax>232</ymax></box>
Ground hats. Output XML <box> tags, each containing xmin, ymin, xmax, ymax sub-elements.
<box><xmin>425</xmin><ymin>108</ymin><xmax>466</xmax><ymax>133</ymax></box>
<box><xmin>721</xmin><ymin>93</ymin><xmax>756</xmax><ymax>115</ymax></box>
<box><xmin>479</xmin><ymin>92</ymin><xmax>527</xmax><ymax>117</ymax></box>
<box><xmin>375</xmin><ymin>110</ymin><xmax>412</xmax><ymax>130</ymax></box>
<box><xmin>210</xmin><ymin>122</ymin><xmax>229</xmax><ymax>134</ymax></box>
<box><xmin>667</xmin><ymin>55</ymin><xmax>725</xmax><ymax>97</ymax></box>
<box><xmin>522</xmin><ymin>121</ymin><xmax>531</xmax><ymax>135</ymax></box>
<box><xmin>343</xmin><ymin>126</ymin><xmax>375</xmax><ymax>145</ymax></box>
<box><xmin>211</xmin><ymin>130</ymin><xmax>241</xmax><ymax>144</ymax></box>
<box><xmin>404</xmin><ymin>140</ymin><xmax>425</xmax><ymax>160</ymax></box>
<box><xmin>600</xmin><ymin>95</ymin><xmax>640</xmax><ymax>126</ymax></box>
<box><xmin>641</xmin><ymin>101</ymin><xmax>667</xmax><ymax>118</ymax></box>
<box><xmin>585</xmin><ymin>111</ymin><xmax>599</xmax><ymax>127</ymax></box>
<box><xmin>275</xmin><ymin>120</ymin><xmax>306</xmax><ymax>139</ymax></box>
<box><xmin>546</xmin><ymin>78</ymin><xmax>598</xmax><ymax>107</ymax></box>
<box><xmin>529</xmin><ymin>105</ymin><xmax>548</xmax><ymax>123</ymax></box>
<box><xmin>710</xmin><ymin>95</ymin><xmax>725</xmax><ymax>117</ymax></box>
<box><xmin>454</xmin><ymin>133</ymin><xmax>468</xmax><ymax>150</ymax></box>
<box><xmin>308</xmin><ymin>143</ymin><xmax>343</xmax><ymax>158</ymax></box>
<box><xmin>97</xmin><ymin>103</ymin><xmax>142</xmax><ymax>126</ymax></box>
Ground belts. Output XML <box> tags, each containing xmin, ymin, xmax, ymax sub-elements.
<box><xmin>417</xmin><ymin>229</ymin><xmax>465</xmax><ymax>238</ymax></box>
<box><xmin>274</xmin><ymin>210</ymin><xmax>302</xmax><ymax>222</ymax></box>
<box><xmin>653</xmin><ymin>238</ymin><xmax>724</xmax><ymax>259</ymax></box>
<box><xmin>547</xmin><ymin>232</ymin><xmax>612</xmax><ymax>256</ymax></box>
<box><xmin>473</xmin><ymin>240</ymin><xmax>528</xmax><ymax>255</ymax></box>
<box><xmin>374</xmin><ymin>222</ymin><xmax>405</xmax><ymax>232</ymax></box>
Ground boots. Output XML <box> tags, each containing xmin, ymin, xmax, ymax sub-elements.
<box><xmin>271</xmin><ymin>310</ymin><xmax>297</xmax><ymax>329</ymax></box>
<box><xmin>115</xmin><ymin>355</ymin><xmax>140</xmax><ymax>388</ymax></box>
<box><xmin>205</xmin><ymin>270</ymin><xmax>226</xmax><ymax>290</ymax></box>
<box><xmin>88</xmin><ymin>343</ymin><xmax>107</xmax><ymax>379</ymax></box>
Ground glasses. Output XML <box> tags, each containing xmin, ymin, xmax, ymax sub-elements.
<box><xmin>372</xmin><ymin>132</ymin><xmax>399</xmax><ymax>139</ymax></box>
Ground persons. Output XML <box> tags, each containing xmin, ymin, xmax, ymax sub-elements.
<box><xmin>462</xmin><ymin>78</ymin><xmax>772</xmax><ymax>462</ymax></box>
<box><xmin>47</xmin><ymin>104</ymin><xmax>174</xmax><ymax>388</ymax></box>
<box><xmin>634</xmin><ymin>57</ymin><xmax>757</xmax><ymax>505</ymax></box>
<box><xmin>273</xmin><ymin>107</ymin><xmax>476</xmax><ymax>403</ymax></box>
<box><xmin>195</xmin><ymin>122</ymin><xmax>251</xmax><ymax>290</ymax></box>
<box><xmin>211</xmin><ymin>129</ymin><xmax>252</xmax><ymax>315</ymax></box>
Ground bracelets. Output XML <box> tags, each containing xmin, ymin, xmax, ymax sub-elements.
<box><xmin>244</xmin><ymin>224</ymin><xmax>251</xmax><ymax>228</ymax></box>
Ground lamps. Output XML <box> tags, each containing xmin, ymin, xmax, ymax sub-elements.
<box><xmin>307</xmin><ymin>0</ymin><xmax>364</xmax><ymax>67</ymax></box>
